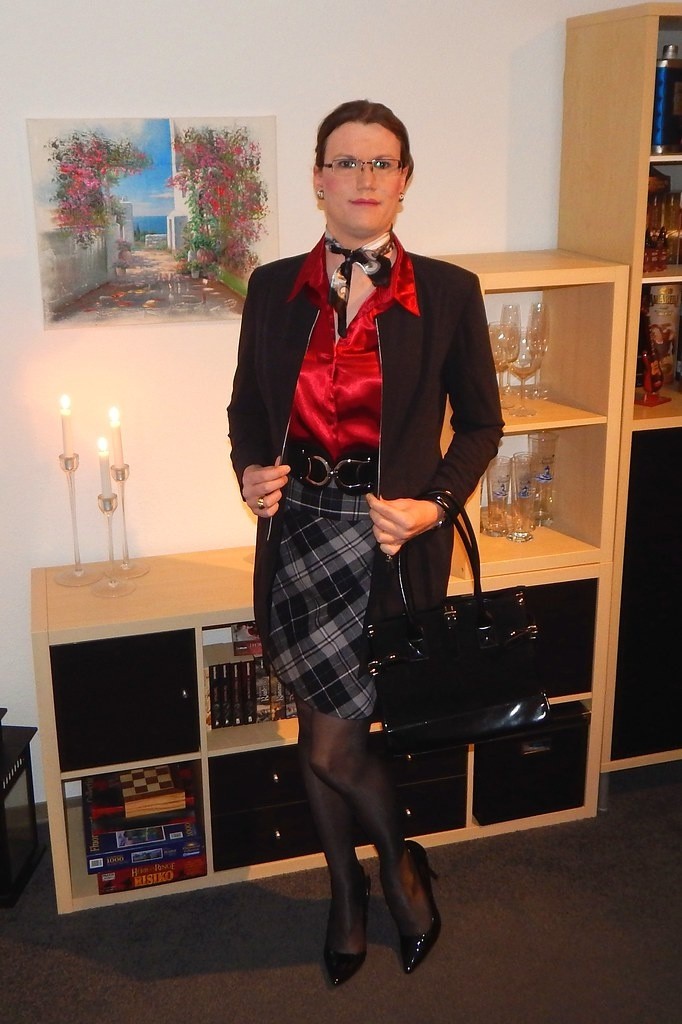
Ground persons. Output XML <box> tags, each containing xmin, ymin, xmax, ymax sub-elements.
<box><xmin>226</xmin><ymin>100</ymin><xmax>504</xmax><ymax>991</ymax></box>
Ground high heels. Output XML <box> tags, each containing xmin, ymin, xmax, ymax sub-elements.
<box><xmin>378</xmin><ymin>838</ymin><xmax>438</xmax><ymax>974</ymax></box>
<box><xmin>323</xmin><ymin>868</ymin><xmax>371</xmax><ymax>986</ymax></box>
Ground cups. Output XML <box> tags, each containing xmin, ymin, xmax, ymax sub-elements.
<box><xmin>480</xmin><ymin>432</ymin><xmax>558</xmax><ymax>542</ymax></box>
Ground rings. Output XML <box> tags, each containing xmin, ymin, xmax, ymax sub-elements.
<box><xmin>258</xmin><ymin>498</ymin><xmax>264</xmax><ymax>510</ymax></box>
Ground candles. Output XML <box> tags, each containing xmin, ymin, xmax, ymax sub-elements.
<box><xmin>59</xmin><ymin>395</ymin><xmax>75</xmax><ymax>459</ymax></box>
<box><xmin>107</xmin><ymin>407</ymin><xmax>124</xmax><ymax>468</ymax></box>
<box><xmin>97</xmin><ymin>437</ymin><xmax>113</xmax><ymax>497</ymax></box>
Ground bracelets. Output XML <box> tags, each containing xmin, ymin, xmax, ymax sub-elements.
<box><xmin>438</xmin><ymin>509</ymin><xmax>446</xmax><ymax>526</ymax></box>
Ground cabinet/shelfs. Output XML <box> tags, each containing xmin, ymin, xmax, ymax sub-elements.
<box><xmin>420</xmin><ymin>249</ymin><xmax>631</xmax><ymax>582</ymax></box>
<box><xmin>30</xmin><ymin>545</ymin><xmax>615</xmax><ymax>919</ymax></box>
<box><xmin>557</xmin><ymin>1</ymin><xmax>682</xmax><ymax>815</ymax></box>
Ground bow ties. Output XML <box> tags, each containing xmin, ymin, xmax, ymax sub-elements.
<box><xmin>325</xmin><ymin>226</ymin><xmax>398</xmax><ymax>339</ymax></box>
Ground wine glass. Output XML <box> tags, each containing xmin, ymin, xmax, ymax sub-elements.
<box><xmin>487</xmin><ymin>302</ymin><xmax>551</xmax><ymax>418</ymax></box>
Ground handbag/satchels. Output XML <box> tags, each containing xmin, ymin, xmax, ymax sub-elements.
<box><xmin>367</xmin><ymin>490</ymin><xmax>552</xmax><ymax>758</ymax></box>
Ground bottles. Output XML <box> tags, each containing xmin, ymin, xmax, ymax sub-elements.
<box><xmin>651</xmin><ymin>44</ymin><xmax>682</xmax><ymax>154</ymax></box>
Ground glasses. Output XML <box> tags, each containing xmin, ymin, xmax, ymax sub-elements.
<box><xmin>317</xmin><ymin>157</ymin><xmax>406</xmax><ymax>178</ymax></box>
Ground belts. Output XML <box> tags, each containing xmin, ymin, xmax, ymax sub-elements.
<box><xmin>279</xmin><ymin>445</ymin><xmax>377</xmax><ymax>494</ymax></box>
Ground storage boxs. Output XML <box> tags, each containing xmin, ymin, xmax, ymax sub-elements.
<box><xmin>80</xmin><ymin>775</ymin><xmax>207</xmax><ymax>896</ymax></box>
